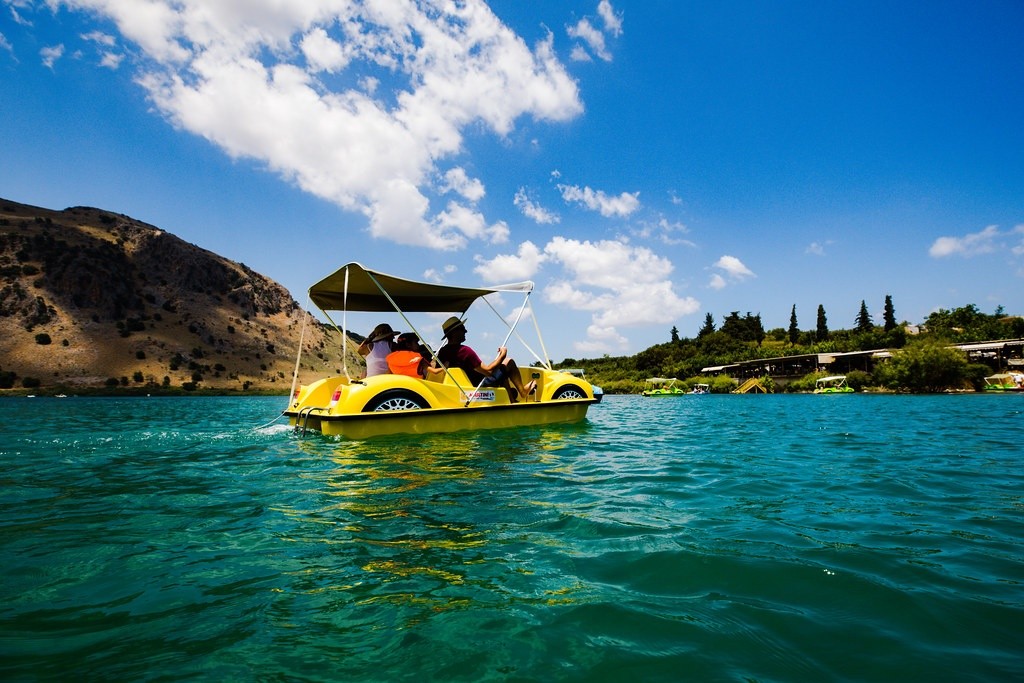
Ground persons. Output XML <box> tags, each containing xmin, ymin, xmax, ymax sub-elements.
<box><xmin>1013</xmin><ymin>374</ymin><xmax>1023</xmax><ymax>387</ymax></box>
<box><xmin>358</xmin><ymin>323</ymin><xmax>449</xmax><ymax>379</ymax></box>
<box><xmin>652</xmin><ymin>383</ymin><xmax>669</xmax><ymax>390</ymax></box>
<box><xmin>817</xmin><ymin>381</ymin><xmax>839</xmax><ymax>390</ymax></box>
<box><xmin>694</xmin><ymin>386</ymin><xmax>701</xmax><ymax>393</ymax></box>
<box><xmin>435</xmin><ymin>316</ymin><xmax>536</xmax><ymax>403</ymax></box>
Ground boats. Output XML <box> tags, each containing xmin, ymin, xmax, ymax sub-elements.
<box><xmin>814</xmin><ymin>376</ymin><xmax>854</xmax><ymax>394</ymax></box>
<box><xmin>559</xmin><ymin>368</ymin><xmax>604</xmax><ymax>404</ymax></box>
<box><xmin>283</xmin><ymin>263</ymin><xmax>598</xmax><ymax>438</ymax></box>
<box><xmin>983</xmin><ymin>374</ymin><xmax>1024</xmax><ymax>394</ymax></box>
<box><xmin>693</xmin><ymin>384</ymin><xmax>711</xmax><ymax>395</ymax></box>
<box><xmin>641</xmin><ymin>377</ymin><xmax>686</xmax><ymax>397</ymax></box>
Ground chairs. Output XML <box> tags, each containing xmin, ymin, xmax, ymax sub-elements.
<box><xmin>426</xmin><ymin>367</ymin><xmax>473</xmax><ymax>388</ymax></box>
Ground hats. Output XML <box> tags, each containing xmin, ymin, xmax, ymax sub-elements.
<box><xmin>442</xmin><ymin>317</ymin><xmax>467</xmax><ymax>340</ymax></box>
<box><xmin>372</xmin><ymin>324</ymin><xmax>401</xmax><ymax>341</ymax></box>
<box><xmin>420</xmin><ymin>344</ymin><xmax>436</xmax><ymax>354</ymax></box>
